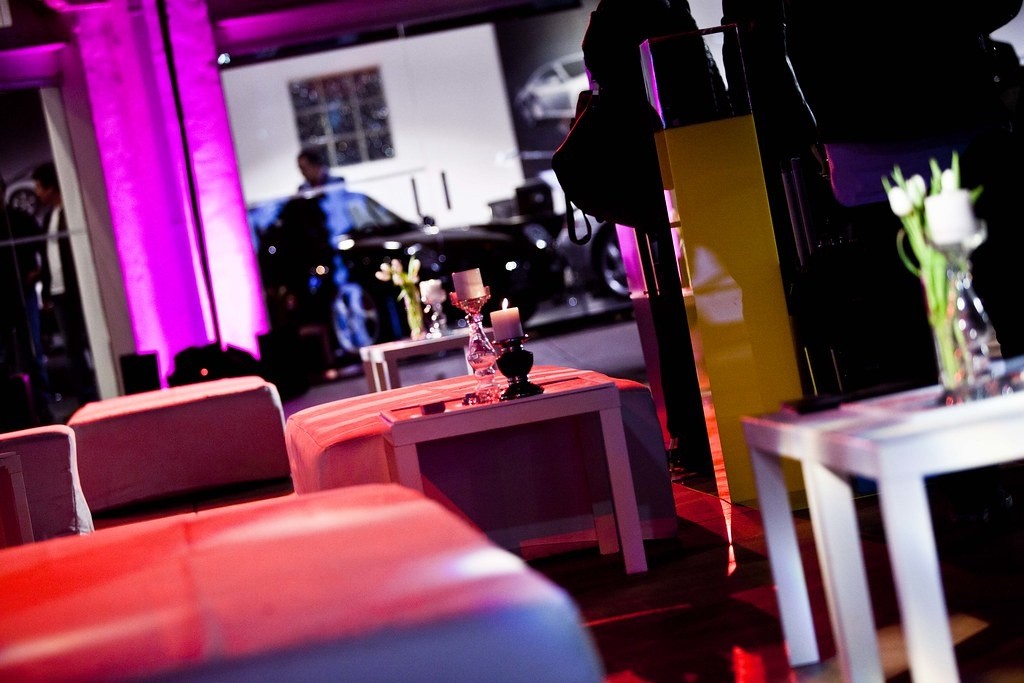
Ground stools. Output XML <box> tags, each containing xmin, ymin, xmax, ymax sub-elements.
<box><xmin>0</xmin><ymin>480</ymin><xmax>602</xmax><ymax>683</ymax></box>
<box><xmin>291</xmin><ymin>365</ymin><xmax>683</xmax><ymax>558</ymax></box>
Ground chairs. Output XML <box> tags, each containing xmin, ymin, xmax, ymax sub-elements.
<box><xmin>0</xmin><ymin>415</ymin><xmax>99</xmax><ymax>553</ymax></box>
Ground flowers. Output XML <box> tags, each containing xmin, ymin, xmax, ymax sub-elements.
<box><xmin>879</xmin><ymin>147</ymin><xmax>1003</xmax><ymax>388</ymax></box>
<box><xmin>375</xmin><ymin>252</ymin><xmax>426</xmax><ymax>340</ymax></box>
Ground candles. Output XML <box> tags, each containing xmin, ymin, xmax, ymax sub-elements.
<box><xmin>419</xmin><ymin>277</ymin><xmax>443</xmax><ymax>300</ymax></box>
<box><xmin>456</xmin><ymin>264</ymin><xmax>484</xmax><ymax>300</ymax></box>
<box><xmin>489</xmin><ymin>294</ymin><xmax>526</xmax><ymax>342</ymax></box>
<box><xmin>921</xmin><ymin>165</ymin><xmax>977</xmax><ymax>244</ymax></box>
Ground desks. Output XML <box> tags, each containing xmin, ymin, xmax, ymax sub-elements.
<box><xmin>727</xmin><ymin>392</ymin><xmax>950</xmax><ymax>683</ymax></box>
<box><xmin>806</xmin><ymin>372</ymin><xmax>1024</xmax><ymax>683</ymax></box>
<box><xmin>64</xmin><ymin>373</ymin><xmax>289</xmax><ymax>513</ymax></box>
<box><xmin>384</xmin><ymin>371</ymin><xmax>649</xmax><ymax>572</ymax></box>
<box><xmin>359</xmin><ymin>327</ymin><xmax>494</xmax><ymax>394</ymax></box>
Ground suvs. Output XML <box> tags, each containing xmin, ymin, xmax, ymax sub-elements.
<box><xmin>247</xmin><ymin>187</ymin><xmax>630</xmax><ymax>364</ymax></box>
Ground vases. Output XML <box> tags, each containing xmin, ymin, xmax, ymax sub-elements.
<box><xmin>463</xmin><ymin>314</ymin><xmax>498</xmax><ymax>376</ymax></box>
<box><xmin>397</xmin><ymin>287</ymin><xmax>429</xmax><ymax>340</ymax></box>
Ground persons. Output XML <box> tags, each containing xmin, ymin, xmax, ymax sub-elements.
<box><xmin>0</xmin><ymin>160</ymin><xmax>99</xmax><ymax>434</ymax></box>
<box><xmin>297</xmin><ymin>150</ymin><xmax>344</xmax><ymax>191</ymax></box>
<box><xmin>583</xmin><ymin>0</ymin><xmax>1024</xmax><ymax>511</ymax></box>
